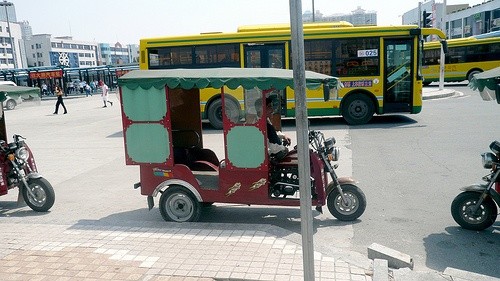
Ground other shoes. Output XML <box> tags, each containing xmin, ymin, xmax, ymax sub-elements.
<box><xmin>53</xmin><ymin>112</ymin><xmax>57</xmax><ymax>114</ymax></box>
<box><xmin>63</xmin><ymin>111</ymin><xmax>67</xmax><ymax>114</ymax></box>
<box><xmin>102</xmin><ymin>106</ymin><xmax>107</xmax><ymax>108</ymax></box>
<box><xmin>110</xmin><ymin>102</ymin><xmax>112</xmax><ymax>106</ymax></box>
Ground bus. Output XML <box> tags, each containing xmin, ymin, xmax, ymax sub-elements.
<box><xmin>139</xmin><ymin>20</ymin><xmax>448</xmax><ymax>126</ymax></box>
<box><xmin>423</xmin><ymin>30</ymin><xmax>500</xmax><ymax>84</ymax></box>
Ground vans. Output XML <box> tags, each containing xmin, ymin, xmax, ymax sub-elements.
<box><xmin>0</xmin><ymin>81</ymin><xmax>23</xmax><ymax>111</ymax></box>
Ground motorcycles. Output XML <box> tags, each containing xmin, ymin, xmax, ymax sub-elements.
<box><xmin>0</xmin><ymin>91</ymin><xmax>55</xmax><ymax>213</ymax></box>
<box><xmin>450</xmin><ymin>66</ymin><xmax>500</xmax><ymax>230</ymax></box>
<box><xmin>118</xmin><ymin>68</ymin><xmax>367</xmax><ymax>222</ymax></box>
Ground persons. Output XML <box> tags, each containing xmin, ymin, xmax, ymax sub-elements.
<box><xmin>101</xmin><ymin>82</ymin><xmax>113</xmax><ymax>107</ymax></box>
<box><xmin>254</xmin><ymin>98</ymin><xmax>291</xmax><ymax>161</ymax></box>
<box><xmin>52</xmin><ymin>86</ymin><xmax>67</xmax><ymax>114</ymax></box>
<box><xmin>35</xmin><ymin>79</ymin><xmax>99</xmax><ymax>97</ymax></box>
